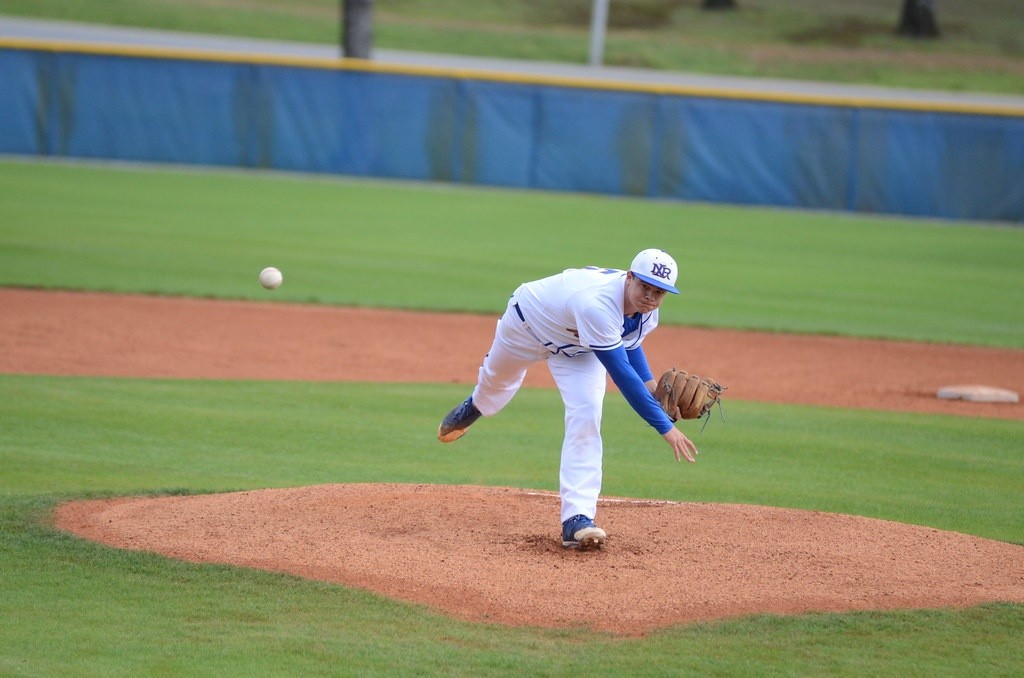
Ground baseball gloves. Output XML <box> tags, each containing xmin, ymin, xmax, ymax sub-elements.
<box><xmin>656</xmin><ymin>368</ymin><xmax>728</xmax><ymax>421</ymax></box>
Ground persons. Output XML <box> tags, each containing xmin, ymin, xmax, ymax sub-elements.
<box><xmin>436</xmin><ymin>249</ymin><xmax>698</xmax><ymax>547</ymax></box>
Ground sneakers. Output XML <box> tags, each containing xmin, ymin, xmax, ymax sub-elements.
<box><xmin>438</xmin><ymin>396</ymin><xmax>482</xmax><ymax>443</ymax></box>
<box><xmin>562</xmin><ymin>515</ymin><xmax>606</xmax><ymax>550</ymax></box>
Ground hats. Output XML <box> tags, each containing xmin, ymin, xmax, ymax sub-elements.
<box><xmin>630</xmin><ymin>248</ymin><xmax>681</xmax><ymax>295</ymax></box>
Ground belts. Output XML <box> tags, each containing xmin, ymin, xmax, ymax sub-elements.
<box><xmin>515</xmin><ymin>304</ymin><xmax>526</xmax><ymax>322</ymax></box>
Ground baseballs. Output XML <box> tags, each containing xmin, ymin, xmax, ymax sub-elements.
<box><xmin>259</xmin><ymin>266</ymin><xmax>282</xmax><ymax>289</ymax></box>
